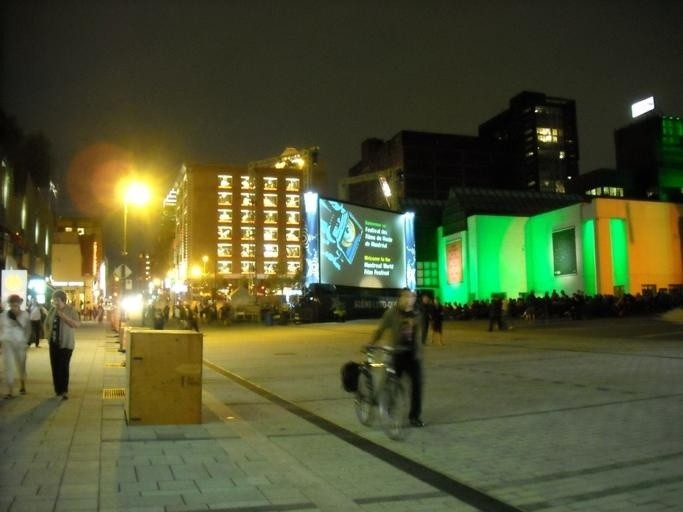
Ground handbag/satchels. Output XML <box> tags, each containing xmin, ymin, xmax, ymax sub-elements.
<box><xmin>341</xmin><ymin>361</ymin><xmax>360</xmax><ymax>393</ymax></box>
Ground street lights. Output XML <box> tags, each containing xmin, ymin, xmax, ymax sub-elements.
<box><xmin>117</xmin><ymin>178</ymin><xmax>154</xmax><ymax>300</ymax></box>
<box><xmin>202</xmin><ymin>253</ymin><xmax>208</xmax><ymax>274</ymax></box>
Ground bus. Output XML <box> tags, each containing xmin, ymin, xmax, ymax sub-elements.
<box><xmin>293</xmin><ymin>282</ymin><xmax>410</xmax><ymax>324</ymax></box>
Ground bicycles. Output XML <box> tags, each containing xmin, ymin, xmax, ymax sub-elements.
<box><xmin>352</xmin><ymin>343</ymin><xmax>411</xmax><ymax>443</ymax></box>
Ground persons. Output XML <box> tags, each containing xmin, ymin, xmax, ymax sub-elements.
<box><xmin>1</xmin><ymin>290</ymin><xmax>230</xmax><ymax>400</ymax></box>
<box><xmin>366</xmin><ymin>287</ymin><xmax>425</xmax><ymax>427</ymax></box>
<box><xmin>418</xmin><ymin>289</ymin><xmax>682</xmax><ymax>346</ymax></box>
